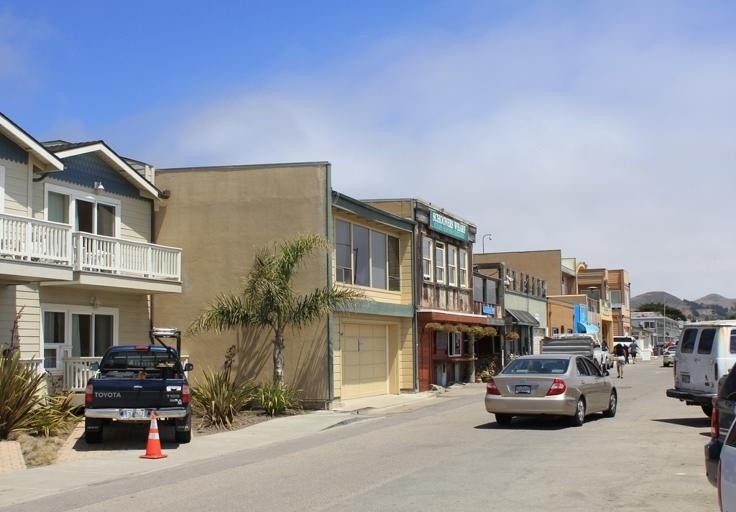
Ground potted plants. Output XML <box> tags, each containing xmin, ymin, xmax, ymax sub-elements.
<box><xmin>481</xmin><ymin>371</ymin><xmax>491</xmax><ymax>382</ymax></box>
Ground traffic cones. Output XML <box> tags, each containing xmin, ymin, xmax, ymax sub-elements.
<box><xmin>139</xmin><ymin>412</ymin><xmax>168</xmax><ymax>459</ymax></box>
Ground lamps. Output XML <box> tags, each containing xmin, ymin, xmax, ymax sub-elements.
<box><xmin>93</xmin><ymin>181</ymin><xmax>104</xmax><ymax>190</ymax></box>
<box><xmin>88</xmin><ymin>296</ymin><xmax>102</xmax><ymax>306</ymax></box>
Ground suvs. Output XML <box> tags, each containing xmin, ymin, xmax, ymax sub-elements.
<box><xmin>702</xmin><ymin>361</ymin><xmax>736</xmax><ymax>488</ymax></box>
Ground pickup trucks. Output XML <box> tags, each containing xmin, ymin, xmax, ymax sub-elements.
<box><xmin>86</xmin><ymin>345</ymin><xmax>193</xmax><ymax>444</ymax></box>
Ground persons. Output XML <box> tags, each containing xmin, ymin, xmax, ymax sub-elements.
<box><xmin>661</xmin><ymin>341</ymin><xmax>669</xmax><ymax>354</ymax></box>
<box><xmin>629</xmin><ymin>339</ymin><xmax>642</xmax><ymax>364</ymax></box>
<box><xmin>602</xmin><ymin>341</ymin><xmax>630</xmax><ymax>364</ymax></box>
<box><xmin>666</xmin><ymin>339</ymin><xmax>675</xmax><ymax>348</ymax></box>
<box><xmin>613</xmin><ymin>343</ymin><xmax>627</xmax><ymax>378</ymax></box>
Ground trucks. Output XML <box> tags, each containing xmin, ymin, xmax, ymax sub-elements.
<box><xmin>540</xmin><ymin>334</ymin><xmax>608</xmax><ymax>374</ymax></box>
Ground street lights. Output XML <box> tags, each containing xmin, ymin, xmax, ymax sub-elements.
<box><xmin>481</xmin><ymin>232</ymin><xmax>492</xmax><ymax>255</ymax></box>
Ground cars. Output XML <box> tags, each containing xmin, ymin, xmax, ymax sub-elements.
<box><xmin>662</xmin><ymin>345</ymin><xmax>677</xmax><ymax>366</ymax></box>
<box><xmin>485</xmin><ymin>354</ymin><xmax>617</xmax><ymax>426</ymax></box>
<box><xmin>653</xmin><ymin>344</ymin><xmax>665</xmax><ymax>355</ymax></box>
<box><xmin>717</xmin><ymin>417</ymin><xmax>735</xmax><ymax>512</ymax></box>
<box><xmin>605</xmin><ymin>348</ymin><xmax>614</xmax><ymax>369</ymax></box>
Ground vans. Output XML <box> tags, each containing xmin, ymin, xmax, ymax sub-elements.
<box><xmin>666</xmin><ymin>319</ymin><xmax>736</xmax><ymax>422</ymax></box>
<box><xmin>613</xmin><ymin>336</ymin><xmax>639</xmax><ymax>353</ymax></box>
